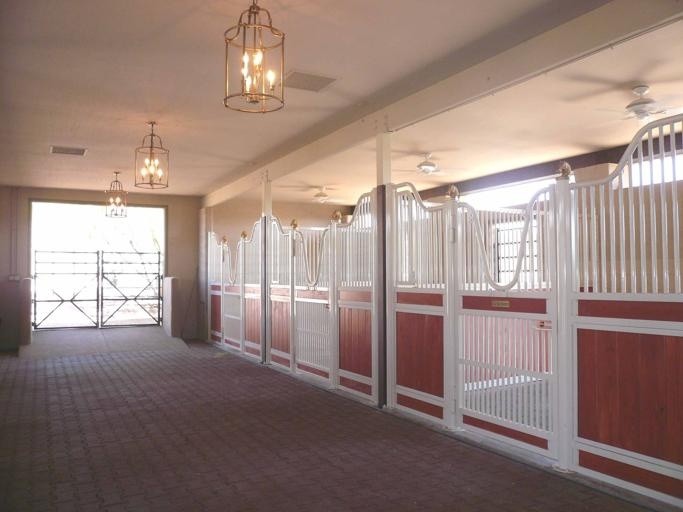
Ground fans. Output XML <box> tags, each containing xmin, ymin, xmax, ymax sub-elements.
<box><xmin>612</xmin><ymin>86</ymin><xmax>683</xmax><ymax>122</ymax></box>
<box><xmin>392</xmin><ymin>153</ymin><xmax>452</xmax><ymax>176</ymax></box>
<box><xmin>313</xmin><ymin>187</ymin><xmax>340</xmax><ymax>204</ymax></box>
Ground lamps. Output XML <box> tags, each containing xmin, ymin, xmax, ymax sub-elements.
<box><xmin>105</xmin><ymin>171</ymin><xmax>128</xmax><ymax>217</ymax></box>
<box><xmin>223</xmin><ymin>0</ymin><xmax>285</xmax><ymax>113</ymax></box>
<box><xmin>134</xmin><ymin>121</ymin><xmax>170</xmax><ymax>189</ymax></box>
